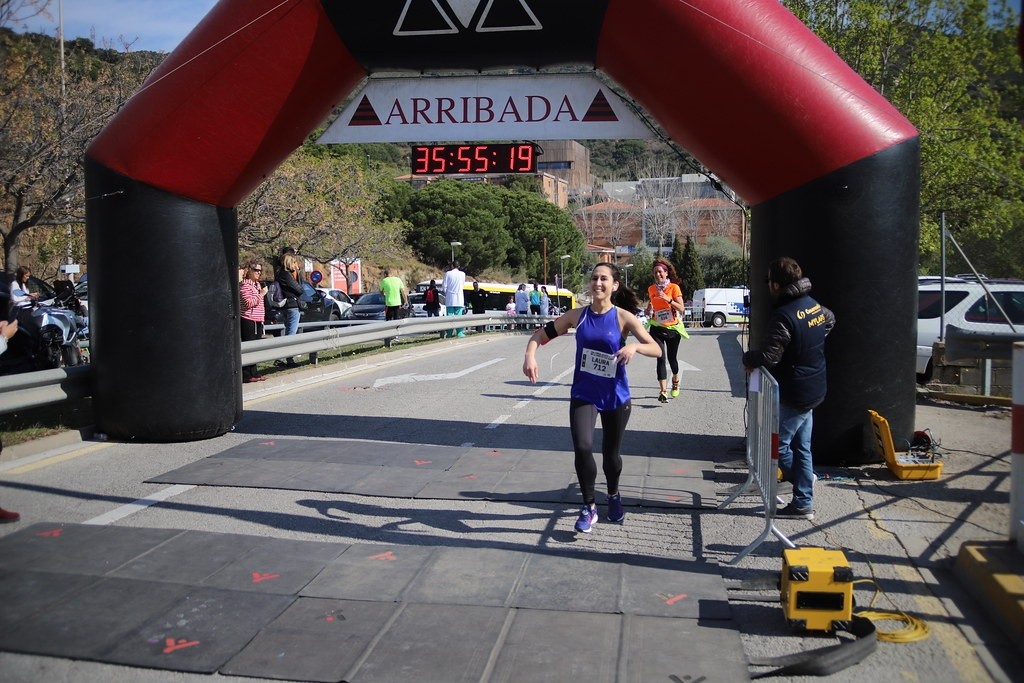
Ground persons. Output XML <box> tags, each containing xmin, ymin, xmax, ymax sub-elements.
<box><xmin>422</xmin><ymin>280</ymin><xmax>440</xmax><ymax>334</ymax></box>
<box><xmin>522</xmin><ymin>261</ymin><xmax>662</xmax><ymax>533</ymax></box>
<box><xmin>53</xmin><ymin>272</ymin><xmax>86</xmax><ymax>317</ymax></box>
<box><xmin>0</xmin><ymin>319</ymin><xmax>21</xmax><ymax>524</ymax></box>
<box><xmin>443</xmin><ymin>261</ymin><xmax>466</xmax><ymax>338</ymax></box>
<box><xmin>742</xmin><ymin>257</ymin><xmax>835</xmax><ymax>519</ymax></box>
<box><xmin>644</xmin><ymin>256</ymin><xmax>689</xmax><ymax>403</ymax></box>
<box><xmin>9</xmin><ymin>266</ymin><xmax>40</xmax><ymax>358</ymax></box>
<box><xmin>506</xmin><ymin>284</ymin><xmax>548</xmax><ymax>332</ymax></box>
<box><xmin>239</xmin><ymin>246</ymin><xmax>304</xmax><ymax>382</ymax></box>
<box><xmin>467</xmin><ymin>281</ymin><xmax>488</xmax><ymax>335</ymax></box>
<box><xmin>379</xmin><ymin>270</ymin><xmax>409</xmax><ymax>321</ymax></box>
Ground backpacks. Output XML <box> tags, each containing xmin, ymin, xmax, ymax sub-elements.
<box><xmin>267</xmin><ymin>277</ymin><xmax>295</xmax><ymax>307</ymax></box>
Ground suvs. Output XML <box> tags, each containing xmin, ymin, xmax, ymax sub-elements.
<box><xmin>918</xmin><ymin>273</ymin><xmax>1024</xmax><ymax>381</ymax></box>
<box><xmin>259</xmin><ymin>280</ymin><xmax>342</xmax><ymax>332</ymax></box>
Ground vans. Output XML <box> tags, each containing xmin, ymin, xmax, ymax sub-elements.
<box><xmin>693</xmin><ymin>285</ymin><xmax>751</xmax><ymax>328</ymax></box>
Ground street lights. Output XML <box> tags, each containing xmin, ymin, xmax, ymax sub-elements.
<box><xmin>451</xmin><ymin>242</ymin><xmax>461</xmax><ymax>263</ymax></box>
<box><xmin>626</xmin><ymin>264</ymin><xmax>633</xmax><ymax>288</ymax></box>
<box><xmin>561</xmin><ymin>255</ymin><xmax>570</xmax><ymax>288</ymax></box>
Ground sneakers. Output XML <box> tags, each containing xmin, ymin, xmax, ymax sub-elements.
<box><xmin>776</xmin><ymin>501</ymin><xmax>814</xmax><ymax>520</ymax></box>
<box><xmin>604</xmin><ymin>491</ymin><xmax>625</xmax><ymax>522</ymax></box>
<box><xmin>811</xmin><ymin>474</ymin><xmax>818</xmax><ymax>489</ymax></box>
<box><xmin>574</xmin><ymin>505</ymin><xmax>598</xmax><ymax>532</ymax></box>
<box><xmin>671</xmin><ymin>376</ymin><xmax>680</xmax><ymax>398</ymax></box>
<box><xmin>658</xmin><ymin>390</ymin><xmax>670</xmax><ymax>403</ymax></box>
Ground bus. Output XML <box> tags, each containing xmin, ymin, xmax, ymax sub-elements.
<box><xmin>416</xmin><ymin>282</ymin><xmax>575</xmax><ymax>314</ymax></box>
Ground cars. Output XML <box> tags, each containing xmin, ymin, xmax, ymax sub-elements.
<box><xmin>316</xmin><ymin>287</ymin><xmax>355</xmax><ymax>319</ymax></box>
<box><xmin>636</xmin><ymin>306</ymin><xmax>692</xmax><ymax>325</ymax></box>
<box><xmin>0</xmin><ymin>269</ymin><xmax>88</xmax><ymax>311</ymax></box>
<box><xmin>408</xmin><ymin>292</ymin><xmax>448</xmax><ymax>316</ymax></box>
<box><xmin>348</xmin><ymin>292</ymin><xmax>412</xmax><ymax>320</ymax></box>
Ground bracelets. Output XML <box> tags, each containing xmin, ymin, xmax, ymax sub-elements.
<box><xmin>669</xmin><ymin>299</ymin><xmax>672</xmax><ymax>303</ymax></box>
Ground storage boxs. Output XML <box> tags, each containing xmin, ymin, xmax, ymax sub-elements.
<box><xmin>869</xmin><ymin>409</ymin><xmax>942</xmax><ymax>479</ymax></box>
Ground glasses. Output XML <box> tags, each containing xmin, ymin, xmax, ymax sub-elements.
<box><xmin>250</xmin><ymin>268</ymin><xmax>263</xmax><ymax>274</ymax></box>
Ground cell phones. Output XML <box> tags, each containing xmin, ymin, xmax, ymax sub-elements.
<box><xmin>8</xmin><ymin>305</ymin><xmax>20</xmax><ymax>324</ymax></box>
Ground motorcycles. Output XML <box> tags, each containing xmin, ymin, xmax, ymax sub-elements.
<box><xmin>11</xmin><ymin>271</ymin><xmax>90</xmax><ymax>367</ymax></box>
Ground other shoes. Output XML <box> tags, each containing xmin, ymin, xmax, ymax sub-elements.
<box><xmin>0</xmin><ymin>507</ymin><xmax>20</xmax><ymax>523</ymax></box>
<box><xmin>446</xmin><ymin>335</ymin><xmax>453</xmax><ymax>339</ymax></box>
<box><xmin>457</xmin><ymin>333</ymin><xmax>465</xmax><ymax>338</ymax></box>
<box><xmin>274</xmin><ymin>360</ymin><xmax>299</xmax><ymax>368</ymax></box>
<box><xmin>243</xmin><ymin>370</ymin><xmax>266</xmax><ymax>381</ymax></box>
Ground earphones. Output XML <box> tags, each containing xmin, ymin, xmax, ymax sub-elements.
<box><xmin>613</xmin><ymin>285</ymin><xmax>616</xmax><ymax>290</ymax></box>
